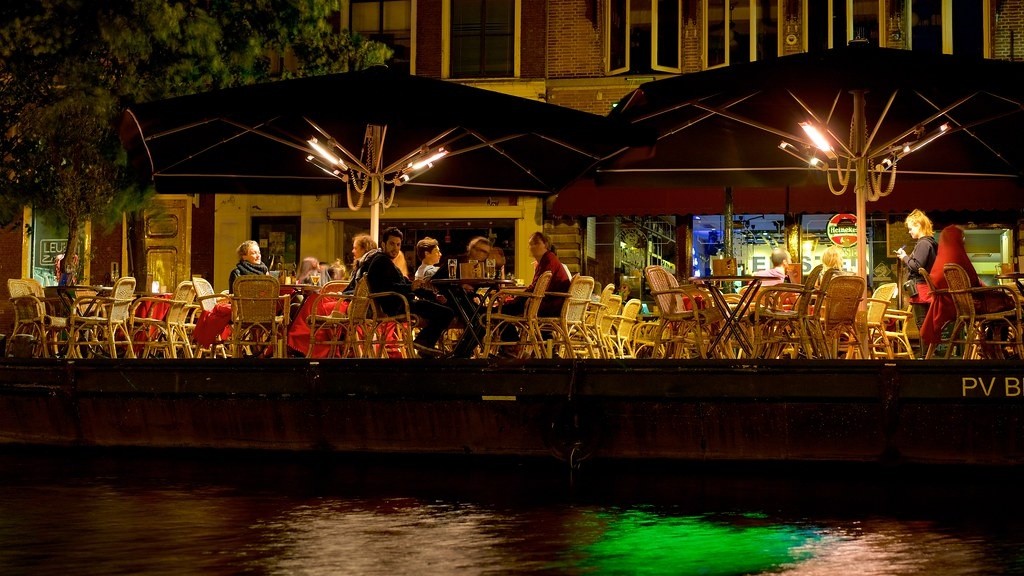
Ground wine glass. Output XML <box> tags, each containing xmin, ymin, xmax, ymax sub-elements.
<box><xmin>311</xmin><ymin>273</ymin><xmax>320</xmax><ymax>286</ymax></box>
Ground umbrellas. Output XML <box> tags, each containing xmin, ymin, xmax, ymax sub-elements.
<box><xmin>125</xmin><ymin>63</ymin><xmax>657</xmax><ymax>356</ymax></box>
<box><xmin>546</xmin><ymin>33</ymin><xmax>1024</xmax><ymax>314</ymax></box>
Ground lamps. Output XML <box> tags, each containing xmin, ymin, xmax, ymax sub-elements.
<box><xmin>777</xmin><ymin>113</ymin><xmax>951</xmax><ymax>202</ymax></box>
<box><xmin>305</xmin><ymin>125</ymin><xmax>451</xmax><ymax>212</ymax></box>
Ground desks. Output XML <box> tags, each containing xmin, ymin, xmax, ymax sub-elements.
<box><xmin>995</xmin><ymin>273</ymin><xmax>1024</xmax><ymax>299</ymax></box>
<box><xmin>132</xmin><ymin>291</ymin><xmax>174</xmax><ymax>360</ymax></box>
<box><xmin>429</xmin><ymin>278</ymin><xmax>515</xmax><ymax>361</ymax></box>
<box><xmin>243</xmin><ymin>284</ymin><xmax>321</xmax><ymax>359</ymax></box>
<box><xmin>688</xmin><ymin>275</ymin><xmax>781</xmax><ymax>360</ymax></box>
<box><xmin>46</xmin><ymin>285</ymin><xmax>111</xmax><ymax>359</ymax></box>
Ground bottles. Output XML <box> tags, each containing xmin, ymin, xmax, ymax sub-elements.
<box><xmin>291</xmin><ymin>271</ymin><xmax>296</xmax><ymax>284</ymax></box>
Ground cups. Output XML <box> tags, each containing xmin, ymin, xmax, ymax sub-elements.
<box><xmin>486</xmin><ymin>259</ymin><xmax>495</xmax><ymax>279</ymax></box>
<box><xmin>1001</xmin><ymin>263</ymin><xmax>1014</xmax><ymax>275</ymax></box>
<box><xmin>448</xmin><ymin>259</ymin><xmax>457</xmax><ymax>279</ymax></box>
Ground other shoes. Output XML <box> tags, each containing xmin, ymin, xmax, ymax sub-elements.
<box><xmin>413</xmin><ymin>340</ymin><xmax>431</xmax><ymax>358</ymax></box>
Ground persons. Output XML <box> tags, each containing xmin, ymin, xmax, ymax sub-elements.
<box><xmin>413</xmin><ymin>234</ymin><xmax>443</xmax><ymax>290</ymax></box>
<box><xmin>748</xmin><ymin>249</ymin><xmax>792</xmax><ymax>324</ymax></box>
<box><xmin>288</xmin><ymin>234</ymin><xmax>376</xmax><ymax>360</ymax></box>
<box><xmin>548</xmin><ymin>244</ymin><xmax>572</xmax><ymax>282</ymax></box>
<box><xmin>367</xmin><ymin>226</ymin><xmax>454</xmax><ymax>357</ymax></box>
<box><xmin>431</xmin><ymin>237</ymin><xmax>494</xmax><ymax>358</ymax></box>
<box><xmin>919</xmin><ymin>226</ymin><xmax>1017</xmax><ymax>357</ymax></box>
<box><xmin>811</xmin><ymin>245</ymin><xmax>843</xmax><ymax>306</ymax></box>
<box><xmin>892</xmin><ymin>209</ymin><xmax>938</xmax><ymax>360</ymax></box>
<box><xmin>226</xmin><ymin>240</ymin><xmax>296</xmax><ymax>313</ymax></box>
<box><xmin>494</xmin><ymin>230</ymin><xmax>571</xmax><ymax>359</ymax></box>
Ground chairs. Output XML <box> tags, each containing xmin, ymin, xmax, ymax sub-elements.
<box><xmin>845</xmin><ymin>283</ymin><xmax>898</xmax><ymax>360</ymax></box>
<box><xmin>942</xmin><ymin>263</ymin><xmax>1024</xmax><ymax>360</ymax></box>
<box><xmin>918</xmin><ymin>268</ymin><xmax>957</xmax><ymax>360</ymax></box>
<box><xmin>5</xmin><ymin>263</ymin><xmax>839</xmax><ymax>361</ymax></box>
<box><xmin>820</xmin><ymin>275</ymin><xmax>867</xmax><ymax>360</ymax></box>
<box><xmin>870</xmin><ymin>304</ymin><xmax>915</xmax><ymax>360</ymax></box>
